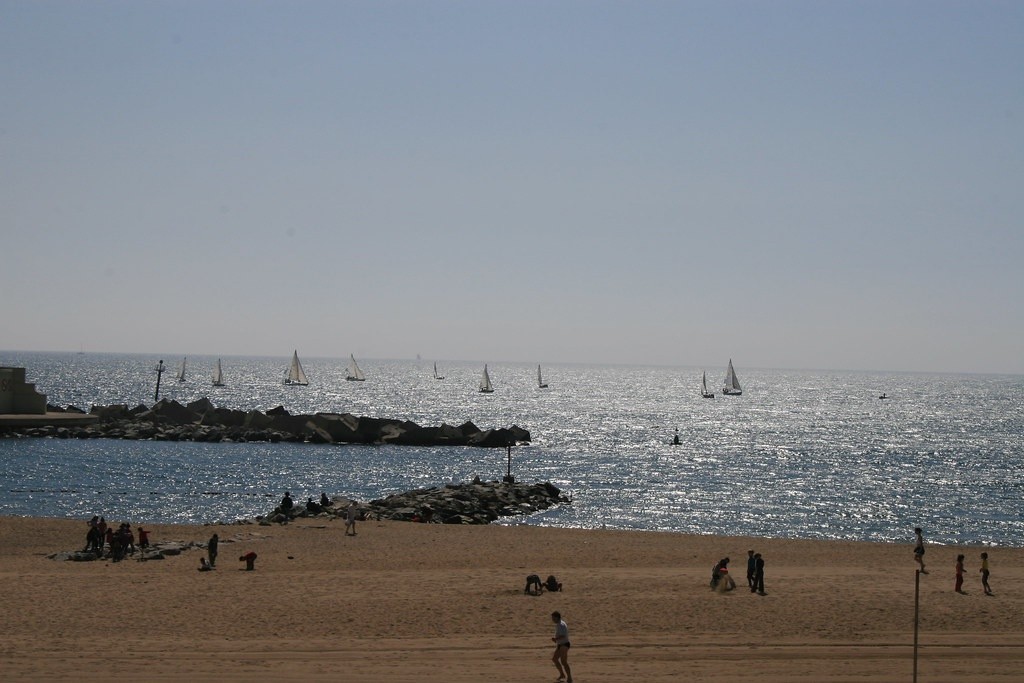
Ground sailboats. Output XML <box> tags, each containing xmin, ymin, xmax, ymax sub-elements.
<box><xmin>701</xmin><ymin>372</ymin><xmax>714</xmax><ymax>398</ymax></box>
<box><xmin>345</xmin><ymin>353</ymin><xmax>366</xmax><ymax>381</ymax></box>
<box><xmin>723</xmin><ymin>359</ymin><xmax>742</xmax><ymax>395</ymax></box>
<box><xmin>285</xmin><ymin>349</ymin><xmax>309</xmax><ymax>385</ymax></box>
<box><xmin>178</xmin><ymin>356</ymin><xmax>187</xmax><ymax>381</ymax></box>
<box><xmin>479</xmin><ymin>363</ymin><xmax>495</xmax><ymax>393</ymax></box>
<box><xmin>434</xmin><ymin>361</ymin><xmax>445</xmax><ymax>379</ymax></box>
<box><xmin>212</xmin><ymin>358</ymin><xmax>225</xmax><ymax>386</ymax></box>
<box><xmin>537</xmin><ymin>364</ymin><xmax>547</xmax><ymax>389</ymax></box>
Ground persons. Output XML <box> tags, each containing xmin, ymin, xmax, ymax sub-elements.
<box><xmin>320</xmin><ymin>493</ymin><xmax>328</xmax><ymax>505</ymax></box>
<box><xmin>955</xmin><ymin>555</ymin><xmax>967</xmax><ymax>592</ymax></box>
<box><xmin>198</xmin><ymin>558</ymin><xmax>211</xmax><ymax>570</ymax></box>
<box><xmin>282</xmin><ymin>493</ymin><xmax>291</xmax><ymax>508</ymax></box>
<box><xmin>551</xmin><ymin>611</ymin><xmax>572</xmax><ymax>683</ymax></box>
<box><xmin>138</xmin><ymin>527</ymin><xmax>150</xmax><ymax>549</ymax></box>
<box><xmin>307</xmin><ymin>498</ymin><xmax>316</xmax><ymax>511</ymax></box>
<box><xmin>239</xmin><ymin>552</ymin><xmax>257</xmax><ymax>569</ymax></box>
<box><xmin>710</xmin><ymin>558</ymin><xmax>729</xmax><ymax>589</ymax></box>
<box><xmin>106</xmin><ymin>522</ymin><xmax>135</xmax><ymax>561</ymax></box>
<box><xmin>524</xmin><ymin>575</ymin><xmax>543</xmax><ymax>594</ymax></box>
<box><xmin>83</xmin><ymin>516</ymin><xmax>107</xmax><ymax>552</ymax></box>
<box><xmin>347</xmin><ymin>501</ymin><xmax>357</xmax><ymax>535</ymax></box>
<box><xmin>747</xmin><ymin>549</ymin><xmax>768</xmax><ymax>595</ymax></box>
<box><xmin>542</xmin><ymin>575</ymin><xmax>562</xmax><ymax>592</ymax></box>
<box><xmin>980</xmin><ymin>553</ymin><xmax>991</xmax><ymax>593</ymax></box>
<box><xmin>914</xmin><ymin>528</ymin><xmax>926</xmax><ymax>572</ymax></box>
<box><xmin>208</xmin><ymin>534</ymin><xmax>218</xmax><ymax>567</ymax></box>
